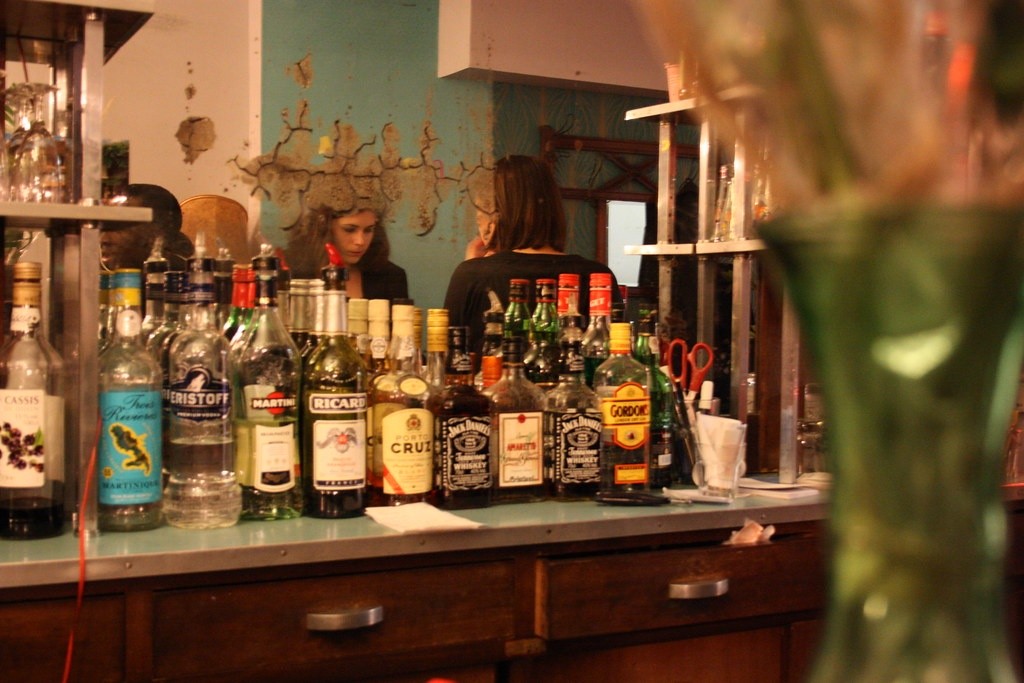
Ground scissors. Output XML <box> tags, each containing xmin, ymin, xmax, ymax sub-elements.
<box><xmin>667</xmin><ymin>338</ymin><xmax>714</xmax><ymax>401</ymax></box>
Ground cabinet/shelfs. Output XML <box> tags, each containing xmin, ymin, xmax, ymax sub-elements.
<box><xmin>1</xmin><ymin>472</ymin><xmax>1024</xmax><ymax>683</ymax></box>
<box><xmin>624</xmin><ymin>48</ymin><xmax>812</xmax><ymax>485</ymax></box>
<box><xmin>0</xmin><ymin>0</ymin><xmax>154</xmax><ymax>540</ymax></box>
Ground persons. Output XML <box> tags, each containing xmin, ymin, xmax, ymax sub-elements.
<box><xmin>444</xmin><ymin>154</ymin><xmax>626</xmax><ymax>357</ymax></box>
<box><xmin>100</xmin><ymin>183</ymin><xmax>183</xmax><ymax>269</ymax></box>
<box><xmin>286</xmin><ymin>202</ymin><xmax>409</xmax><ymax>300</ymax></box>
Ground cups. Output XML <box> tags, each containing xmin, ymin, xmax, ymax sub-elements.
<box><xmin>670</xmin><ymin>398</ymin><xmax>720</xmax><ymax>485</ymax></box>
<box><xmin>693</xmin><ymin>442</ymin><xmax>746</xmax><ymax>499</ymax></box>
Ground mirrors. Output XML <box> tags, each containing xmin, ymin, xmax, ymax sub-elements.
<box><xmin>4</xmin><ymin>0</ymin><xmax>757</xmax><ymax>409</ymax></box>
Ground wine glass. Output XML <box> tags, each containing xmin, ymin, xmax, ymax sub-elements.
<box><xmin>0</xmin><ymin>82</ymin><xmax>72</xmax><ymax>205</ymax></box>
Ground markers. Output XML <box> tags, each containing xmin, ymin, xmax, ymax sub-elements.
<box><xmin>699</xmin><ymin>380</ymin><xmax>714</xmax><ymax>414</ymax></box>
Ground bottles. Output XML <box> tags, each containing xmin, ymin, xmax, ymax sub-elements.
<box><xmin>0</xmin><ymin>261</ymin><xmax>67</xmax><ymax>541</ymax></box>
<box><xmin>796</xmin><ymin>383</ymin><xmax>827</xmax><ymax>475</ymax></box>
<box><xmin>712</xmin><ymin>156</ymin><xmax>771</xmax><ymax>241</ymax></box>
<box><xmin>101</xmin><ymin>254</ymin><xmax>674</xmax><ymax>538</ymax></box>
